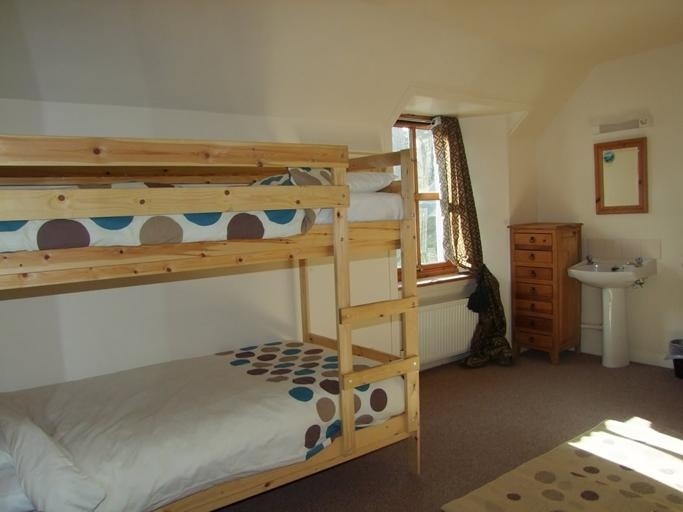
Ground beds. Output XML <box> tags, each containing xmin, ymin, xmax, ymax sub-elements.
<box><xmin>0</xmin><ymin>131</ymin><xmax>421</xmax><ymax>511</ymax></box>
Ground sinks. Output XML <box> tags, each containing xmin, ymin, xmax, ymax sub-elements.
<box><xmin>567</xmin><ymin>257</ymin><xmax>657</xmax><ymax>289</ymax></box>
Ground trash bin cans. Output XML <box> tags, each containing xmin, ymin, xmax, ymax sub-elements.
<box><xmin>664</xmin><ymin>339</ymin><xmax>683</xmax><ymax>378</ymax></box>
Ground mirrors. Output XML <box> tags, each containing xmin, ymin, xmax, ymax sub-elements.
<box><xmin>593</xmin><ymin>136</ymin><xmax>649</xmax><ymax>215</ymax></box>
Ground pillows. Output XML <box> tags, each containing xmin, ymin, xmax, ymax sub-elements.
<box><xmin>345</xmin><ymin>171</ymin><xmax>401</xmax><ymax>193</ymax></box>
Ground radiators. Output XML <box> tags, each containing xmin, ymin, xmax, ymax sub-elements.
<box><xmin>419</xmin><ymin>298</ymin><xmax>478</xmax><ymax>367</ymax></box>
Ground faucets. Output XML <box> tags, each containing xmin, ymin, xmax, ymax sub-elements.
<box><xmin>586</xmin><ymin>255</ymin><xmax>593</xmax><ymax>265</ymax></box>
<box><xmin>626</xmin><ymin>257</ymin><xmax>643</xmax><ymax>267</ymax></box>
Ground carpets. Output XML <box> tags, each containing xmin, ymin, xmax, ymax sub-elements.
<box><xmin>440</xmin><ymin>420</ymin><xmax>683</xmax><ymax>511</ymax></box>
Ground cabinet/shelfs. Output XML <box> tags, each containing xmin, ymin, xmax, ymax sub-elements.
<box><xmin>507</xmin><ymin>221</ymin><xmax>583</xmax><ymax>367</ymax></box>
